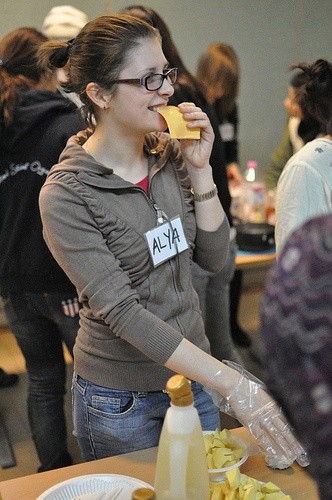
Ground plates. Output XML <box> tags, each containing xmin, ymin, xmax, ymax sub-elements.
<box><xmin>35</xmin><ymin>472</ymin><xmax>155</xmax><ymax>500</ymax></box>
<box><xmin>201</xmin><ymin>430</ymin><xmax>248</xmax><ymax>473</ymax></box>
<box><xmin>209</xmin><ymin>473</ymin><xmax>292</xmax><ymax>500</ymax></box>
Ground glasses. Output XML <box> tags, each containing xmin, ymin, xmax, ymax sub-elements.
<box><xmin>108</xmin><ymin>67</ymin><xmax>178</xmax><ymax>91</ymax></box>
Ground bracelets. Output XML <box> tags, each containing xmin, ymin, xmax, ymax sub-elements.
<box><xmin>190</xmin><ymin>185</ymin><xmax>218</xmax><ymax>202</ymax></box>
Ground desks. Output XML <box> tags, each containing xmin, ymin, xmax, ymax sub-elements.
<box><xmin>0</xmin><ymin>425</ymin><xmax>321</xmax><ymax>500</ymax></box>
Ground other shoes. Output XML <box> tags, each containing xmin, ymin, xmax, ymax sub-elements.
<box><xmin>0</xmin><ymin>367</ymin><xmax>19</xmax><ymax>389</ymax></box>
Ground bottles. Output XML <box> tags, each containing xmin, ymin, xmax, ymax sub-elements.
<box><xmin>241</xmin><ymin>159</ymin><xmax>264</xmax><ymax>222</ymax></box>
<box><xmin>152</xmin><ymin>374</ymin><xmax>209</xmax><ymax>500</ymax></box>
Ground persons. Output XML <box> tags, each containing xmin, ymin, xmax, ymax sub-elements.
<box><xmin>0</xmin><ymin>6</ymin><xmax>332</xmax><ymax>472</ymax></box>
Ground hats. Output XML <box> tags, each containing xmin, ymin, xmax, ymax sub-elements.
<box><xmin>42</xmin><ymin>5</ymin><xmax>89</xmax><ymax>29</ymax></box>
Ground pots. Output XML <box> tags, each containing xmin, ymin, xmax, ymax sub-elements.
<box><xmin>230</xmin><ymin>214</ymin><xmax>275</xmax><ymax>252</ymax></box>
